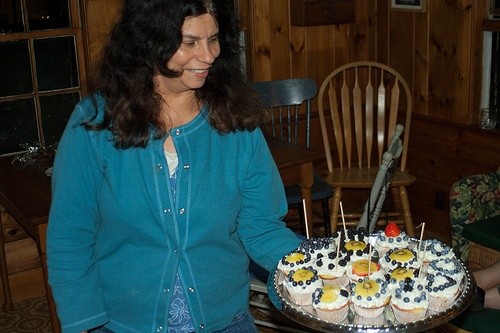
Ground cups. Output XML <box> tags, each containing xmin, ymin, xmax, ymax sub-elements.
<box><xmin>478</xmin><ymin>108</ymin><xmax>497</xmax><ymax>130</ymax></box>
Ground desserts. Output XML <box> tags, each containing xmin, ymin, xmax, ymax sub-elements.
<box><xmin>277</xmin><ymin>222</ymin><xmax>464</xmax><ymax>323</ymax></box>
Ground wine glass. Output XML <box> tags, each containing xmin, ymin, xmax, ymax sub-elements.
<box><xmin>45</xmin><ymin>136</ymin><xmax>60</xmax><ymax>177</ymax></box>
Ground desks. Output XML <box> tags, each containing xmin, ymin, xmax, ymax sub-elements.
<box><xmin>1</xmin><ymin>137</ymin><xmax>325</xmax><ymax>333</ymax></box>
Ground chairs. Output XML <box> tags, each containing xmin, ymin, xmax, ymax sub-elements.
<box><xmin>450</xmin><ymin>167</ymin><xmax>500</xmax><ymax>268</ymax></box>
<box><xmin>317</xmin><ymin>61</ymin><xmax>414</xmax><ymax>237</ymax></box>
<box><xmin>247</xmin><ymin>78</ymin><xmax>333</xmax><ymax>238</ymax></box>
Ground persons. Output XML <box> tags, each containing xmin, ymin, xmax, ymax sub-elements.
<box><xmin>46</xmin><ymin>0</ymin><xmax>305</xmax><ymax>333</ymax></box>
<box><xmin>470</xmin><ymin>259</ymin><xmax>500</xmax><ymax>311</ymax></box>
